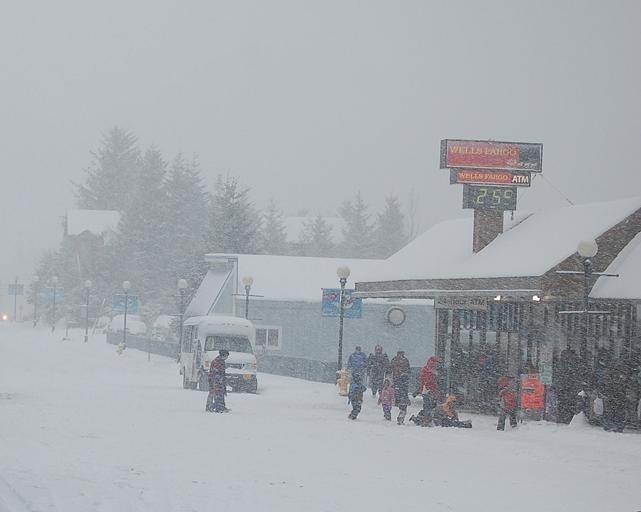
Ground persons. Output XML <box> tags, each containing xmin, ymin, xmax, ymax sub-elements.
<box><xmin>347</xmin><ymin>345</ymin><xmax>641</xmax><ymax>432</ymax></box>
<box><xmin>209</xmin><ymin>374</ymin><xmax>226</xmax><ymax>414</ymax></box>
<box><xmin>205</xmin><ymin>349</ymin><xmax>232</xmax><ymax>413</ymax></box>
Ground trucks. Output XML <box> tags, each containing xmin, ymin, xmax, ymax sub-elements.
<box><xmin>180</xmin><ymin>314</ymin><xmax>258</xmax><ymax>394</ymax></box>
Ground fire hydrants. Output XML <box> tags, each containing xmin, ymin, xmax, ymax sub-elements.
<box><xmin>335</xmin><ymin>367</ymin><xmax>350</xmax><ymax>397</ymax></box>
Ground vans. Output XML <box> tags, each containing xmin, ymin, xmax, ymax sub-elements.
<box><xmin>149</xmin><ymin>315</ymin><xmax>179</xmax><ymax>355</ymax></box>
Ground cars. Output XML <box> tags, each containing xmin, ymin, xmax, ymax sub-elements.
<box><xmin>105</xmin><ymin>315</ymin><xmax>145</xmax><ymax>349</ymax></box>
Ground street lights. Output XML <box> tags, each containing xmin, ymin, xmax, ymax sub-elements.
<box><xmin>122</xmin><ymin>280</ymin><xmax>131</xmax><ymax>349</ymax></box>
<box><xmin>49</xmin><ymin>276</ymin><xmax>60</xmax><ymax>332</ymax></box>
<box><xmin>177</xmin><ymin>278</ymin><xmax>187</xmax><ymax>358</ymax></box>
<box><xmin>335</xmin><ymin>265</ymin><xmax>351</xmax><ymax>384</ymax></box>
<box><xmin>82</xmin><ymin>280</ymin><xmax>92</xmax><ymax>341</ymax></box>
<box><xmin>577</xmin><ymin>234</ymin><xmax>598</xmax><ymax>417</ymax></box>
<box><xmin>242</xmin><ymin>275</ymin><xmax>252</xmax><ymax>319</ymax></box>
<box><xmin>31</xmin><ymin>274</ymin><xmax>40</xmax><ymax>325</ymax></box>
<box><xmin>12</xmin><ymin>272</ymin><xmax>19</xmax><ymax>321</ymax></box>
